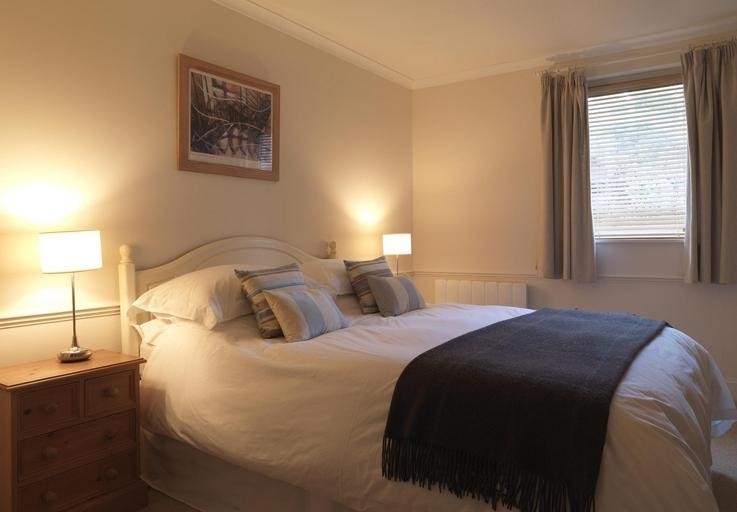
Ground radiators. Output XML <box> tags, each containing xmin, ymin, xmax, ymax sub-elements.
<box><xmin>432</xmin><ymin>280</ymin><xmax>529</xmax><ymax>308</ymax></box>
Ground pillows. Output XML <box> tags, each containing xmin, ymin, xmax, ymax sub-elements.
<box><xmin>133</xmin><ymin>255</ymin><xmax>428</xmax><ymax>342</ymax></box>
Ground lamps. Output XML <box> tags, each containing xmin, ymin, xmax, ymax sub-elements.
<box><xmin>384</xmin><ymin>233</ymin><xmax>412</xmax><ymax>277</ymax></box>
<box><xmin>39</xmin><ymin>230</ymin><xmax>104</xmax><ymax>364</ymax></box>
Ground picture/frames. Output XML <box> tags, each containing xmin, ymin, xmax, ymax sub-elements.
<box><xmin>175</xmin><ymin>51</ymin><xmax>282</xmax><ymax>182</ymax></box>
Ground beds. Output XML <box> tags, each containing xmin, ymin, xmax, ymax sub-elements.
<box><xmin>118</xmin><ymin>236</ymin><xmax>714</xmax><ymax>511</ymax></box>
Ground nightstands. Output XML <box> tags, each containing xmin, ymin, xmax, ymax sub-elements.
<box><xmin>1</xmin><ymin>350</ymin><xmax>149</xmax><ymax>512</ymax></box>
